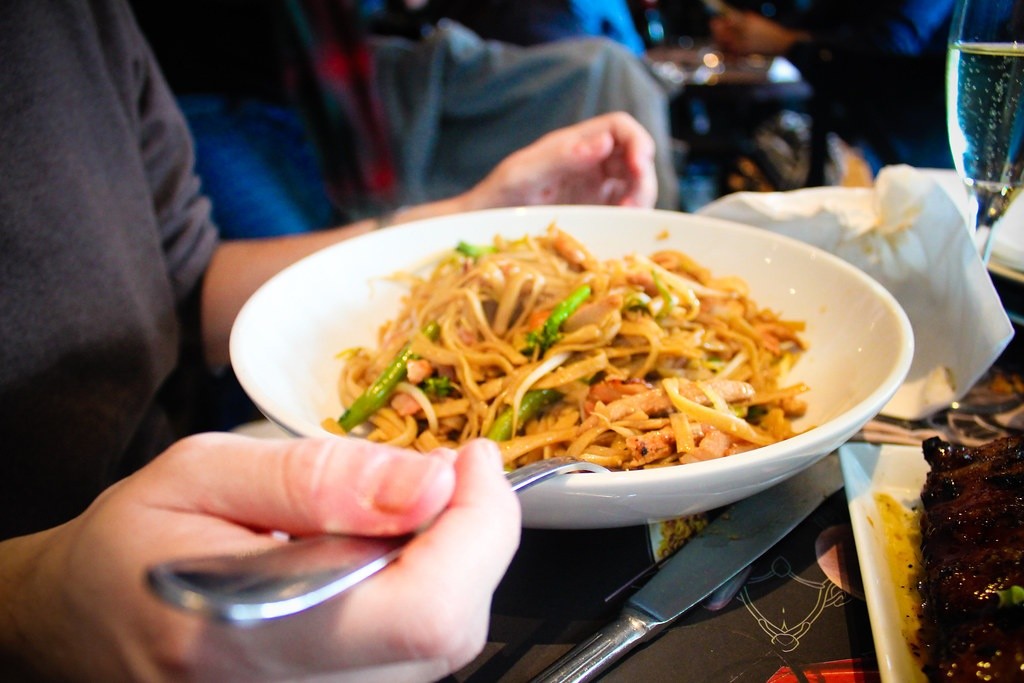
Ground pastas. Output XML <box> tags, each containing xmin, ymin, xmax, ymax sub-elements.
<box><xmin>333</xmin><ymin>224</ymin><xmax>813</xmax><ymax>472</ymax></box>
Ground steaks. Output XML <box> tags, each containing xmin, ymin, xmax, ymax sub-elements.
<box><xmin>914</xmin><ymin>432</ymin><xmax>1024</xmax><ymax>683</ymax></box>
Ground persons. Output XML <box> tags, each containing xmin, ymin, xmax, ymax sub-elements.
<box><xmin>352</xmin><ymin>0</ymin><xmax>1021</xmax><ymax>212</ymax></box>
<box><xmin>0</xmin><ymin>1</ymin><xmax>660</xmax><ymax>683</ymax></box>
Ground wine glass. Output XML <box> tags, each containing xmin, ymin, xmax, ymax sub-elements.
<box><xmin>947</xmin><ymin>0</ymin><xmax>1024</xmax><ymax>412</ymax></box>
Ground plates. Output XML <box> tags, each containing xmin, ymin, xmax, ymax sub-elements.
<box><xmin>230</xmin><ymin>204</ymin><xmax>914</xmax><ymax>529</ymax></box>
<box><xmin>839</xmin><ymin>442</ymin><xmax>931</xmax><ymax>683</ymax></box>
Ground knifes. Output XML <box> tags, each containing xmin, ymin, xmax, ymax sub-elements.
<box><xmin>530</xmin><ymin>450</ymin><xmax>837</xmax><ymax>683</ymax></box>
